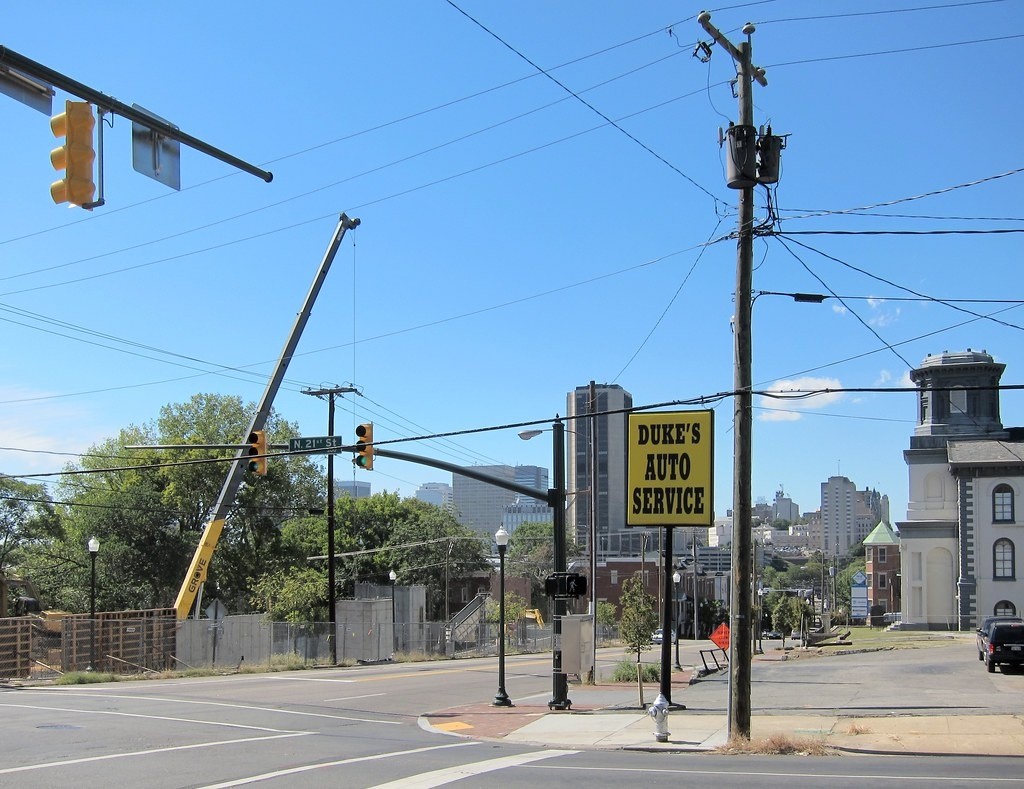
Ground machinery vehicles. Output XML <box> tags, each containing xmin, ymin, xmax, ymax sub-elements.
<box><xmin>525</xmin><ymin>608</ymin><xmax>546</xmax><ymax>630</ymax></box>
<box><xmin>4</xmin><ymin>577</ymin><xmax>73</xmax><ymax>666</ymax></box>
<box><xmin>170</xmin><ymin>211</ymin><xmax>363</xmax><ymax>622</ymax></box>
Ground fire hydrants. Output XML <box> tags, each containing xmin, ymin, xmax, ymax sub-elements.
<box><xmin>648</xmin><ymin>691</ymin><xmax>671</xmax><ymax>742</ymax></box>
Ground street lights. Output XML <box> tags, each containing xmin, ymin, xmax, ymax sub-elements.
<box><xmin>86</xmin><ymin>535</ymin><xmax>101</xmax><ymax>672</ymax></box>
<box><xmin>757</xmin><ymin>587</ymin><xmax>763</xmax><ymax>654</ymax></box>
<box><xmin>518</xmin><ymin>381</ymin><xmax>597</xmax><ymax>686</ymax></box>
<box><xmin>816</xmin><ymin>549</ymin><xmax>824</xmax><ymax>614</ymax></box>
<box><xmin>492</xmin><ymin>522</ymin><xmax>513</xmax><ymax>706</ymax></box>
<box><xmin>673</xmin><ymin>570</ymin><xmax>684</xmax><ymax>671</ymax></box>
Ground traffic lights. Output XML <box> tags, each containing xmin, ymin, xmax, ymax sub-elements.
<box><xmin>355</xmin><ymin>420</ymin><xmax>374</xmax><ymax>471</ymax></box>
<box><xmin>248</xmin><ymin>430</ymin><xmax>268</xmax><ymax>475</ymax></box>
<box><xmin>48</xmin><ymin>98</ymin><xmax>94</xmax><ymax>208</ymax></box>
<box><xmin>564</xmin><ymin>573</ymin><xmax>588</xmax><ymax>595</ymax></box>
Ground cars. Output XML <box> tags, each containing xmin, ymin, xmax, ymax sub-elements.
<box><xmin>769</xmin><ymin>630</ymin><xmax>782</xmax><ymax>640</ymax></box>
<box><xmin>651</xmin><ymin>628</ymin><xmax>676</xmax><ymax>646</ymax></box>
<box><xmin>976</xmin><ymin>616</ymin><xmax>1024</xmax><ymax>673</ymax></box>
<box><xmin>791</xmin><ymin>628</ymin><xmax>819</xmax><ymax>640</ymax></box>
